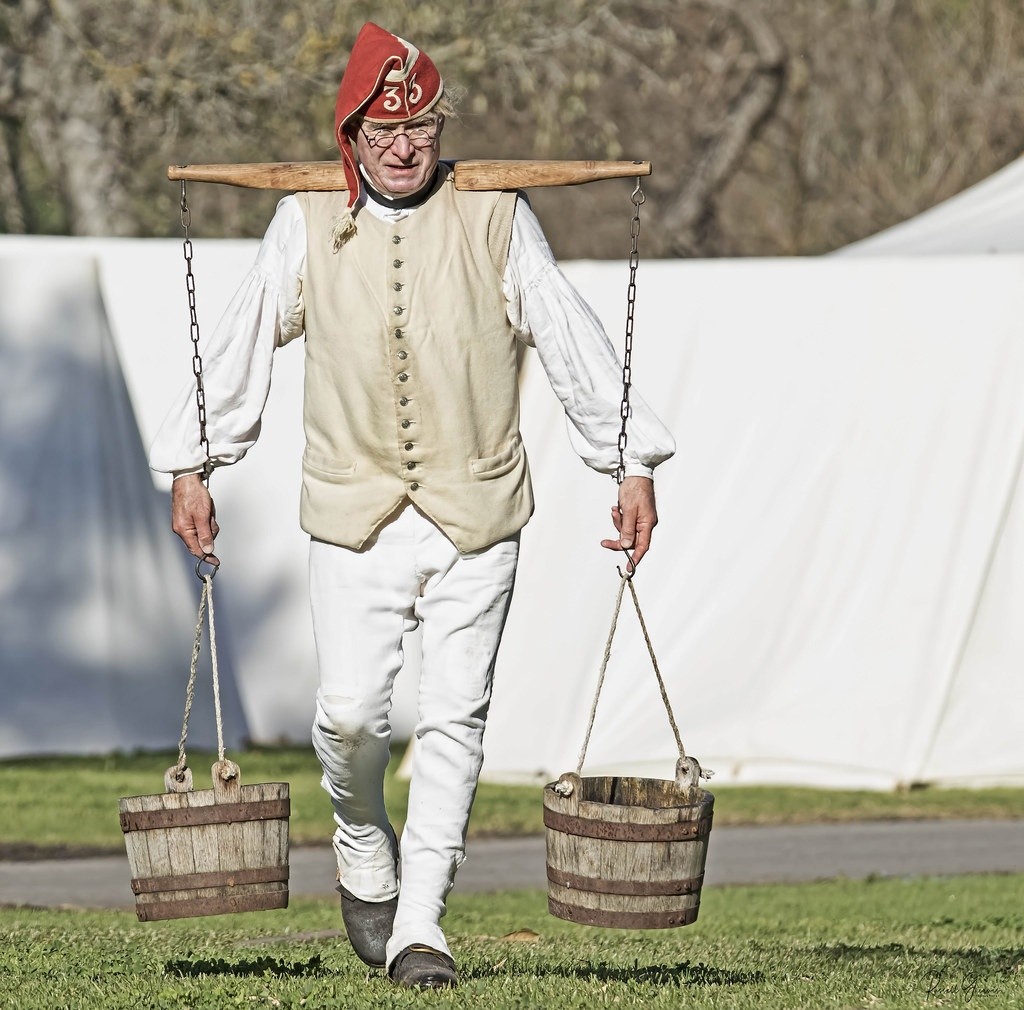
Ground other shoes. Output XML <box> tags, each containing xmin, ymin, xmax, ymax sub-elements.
<box><xmin>390</xmin><ymin>943</ymin><xmax>458</xmax><ymax>987</ymax></box>
<box><xmin>332</xmin><ymin>823</ymin><xmax>399</xmax><ymax>968</ymax></box>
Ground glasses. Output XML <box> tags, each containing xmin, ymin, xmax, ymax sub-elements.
<box><xmin>357</xmin><ymin>122</ymin><xmax>438</xmax><ymax>148</ymax></box>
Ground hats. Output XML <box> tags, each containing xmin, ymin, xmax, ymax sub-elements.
<box><xmin>328</xmin><ymin>22</ymin><xmax>444</xmax><ymax>253</ymax></box>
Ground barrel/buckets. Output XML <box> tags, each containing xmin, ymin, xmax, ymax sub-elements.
<box><xmin>542</xmin><ymin>774</ymin><xmax>715</xmax><ymax>929</ymax></box>
<box><xmin>119</xmin><ymin>782</ymin><xmax>291</xmax><ymax>921</ymax></box>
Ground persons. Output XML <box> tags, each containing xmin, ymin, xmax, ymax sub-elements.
<box><xmin>148</xmin><ymin>22</ymin><xmax>676</xmax><ymax>993</ymax></box>
